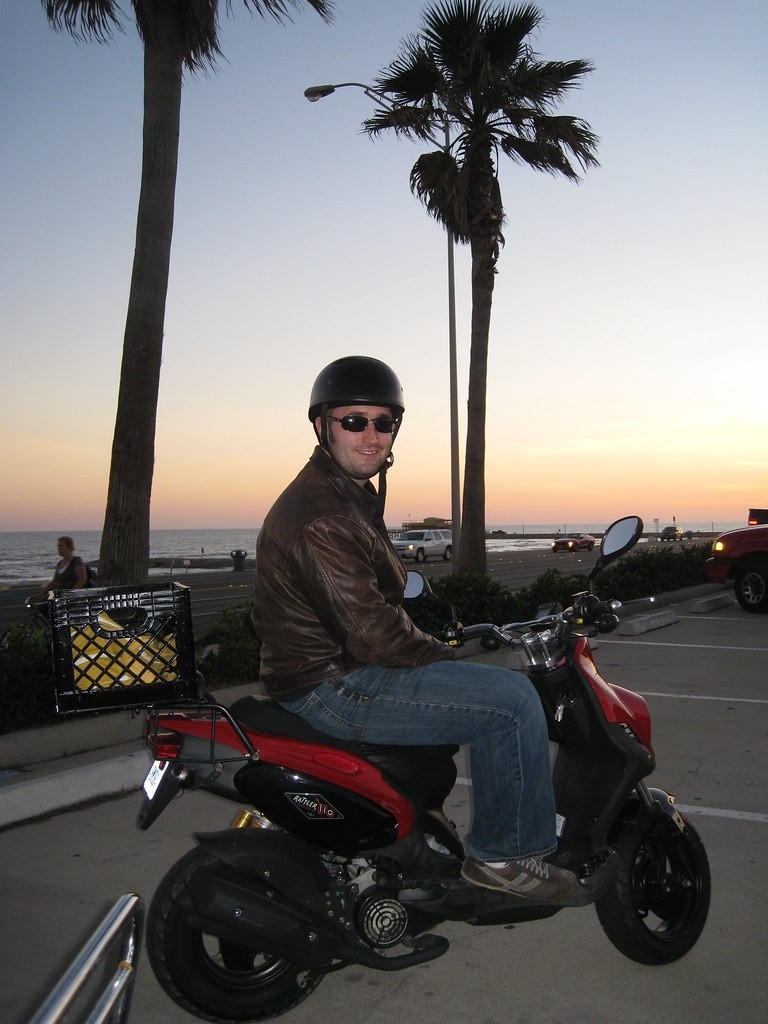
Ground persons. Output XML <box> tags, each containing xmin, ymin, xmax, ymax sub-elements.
<box><xmin>252</xmin><ymin>356</ymin><xmax>578</xmax><ymax>902</ymax></box>
<box><xmin>34</xmin><ymin>536</ymin><xmax>87</xmax><ymax>600</ymax></box>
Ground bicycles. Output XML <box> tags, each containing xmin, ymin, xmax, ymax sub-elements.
<box><xmin>0</xmin><ymin>597</ymin><xmax>57</xmax><ymax>650</ymax></box>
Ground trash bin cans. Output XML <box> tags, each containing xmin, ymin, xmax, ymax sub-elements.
<box><xmin>230</xmin><ymin>550</ymin><xmax>248</xmax><ymax>571</ymax></box>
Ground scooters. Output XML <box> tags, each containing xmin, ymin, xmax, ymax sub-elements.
<box><xmin>136</xmin><ymin>515</ymin><xmax>713</xmax><ymax>1024</ymax></box>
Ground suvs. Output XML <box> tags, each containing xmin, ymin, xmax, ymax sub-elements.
<box><xmin>711</xmin><ymin>524</ymin><xmax>768</xmax><ymax>612</ymax></box>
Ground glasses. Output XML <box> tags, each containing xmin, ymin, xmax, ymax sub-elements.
<box><xmin>325</xmin><ymin>414</ymin><xmax>399</xmax><ymax>433</ymax></box>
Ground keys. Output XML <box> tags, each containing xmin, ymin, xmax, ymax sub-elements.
<box><xmin>554</xmin><ymin>698</ymin><xmax>567</xmax><ymax>721</ymax></box>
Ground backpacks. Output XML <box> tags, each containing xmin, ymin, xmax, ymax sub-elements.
<box><xmin>58</xmin><ymin>556</ymin><xmax>97</xmax><ymax>588</ymax></box>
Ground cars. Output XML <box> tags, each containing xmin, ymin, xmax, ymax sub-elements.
<box><xmin>551</xmin><ymin>533</ymin><xmax>595</xmax><ymax>552</ymax></box>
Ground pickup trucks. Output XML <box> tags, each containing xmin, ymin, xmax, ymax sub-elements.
<box><xmin>392</xmin><ymin>529</ymin><xmax>453</xmax><ymax>562</ymax></box>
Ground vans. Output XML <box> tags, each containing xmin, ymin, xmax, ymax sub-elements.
<box><xmin>662</xmin><ymin>526</ymin><xmax>683</xmax><ymax>541</ymax></box>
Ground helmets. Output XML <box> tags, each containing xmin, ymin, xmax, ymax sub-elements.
<box><xmin>308</xmin><ymin>356</ymin><xmax>405</xmax><ymax>424</ymax></box>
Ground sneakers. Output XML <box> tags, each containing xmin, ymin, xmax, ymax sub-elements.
<box><xmin>461</xmin><ymin>846</ymin><xmax>577</xmax><ymax>902</ymax></box>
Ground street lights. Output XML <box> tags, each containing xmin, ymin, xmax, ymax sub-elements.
<box><xmin>304</xmin><ymin>83</ymin><xmax>461</xmax><ymax>579</ymax></box>
